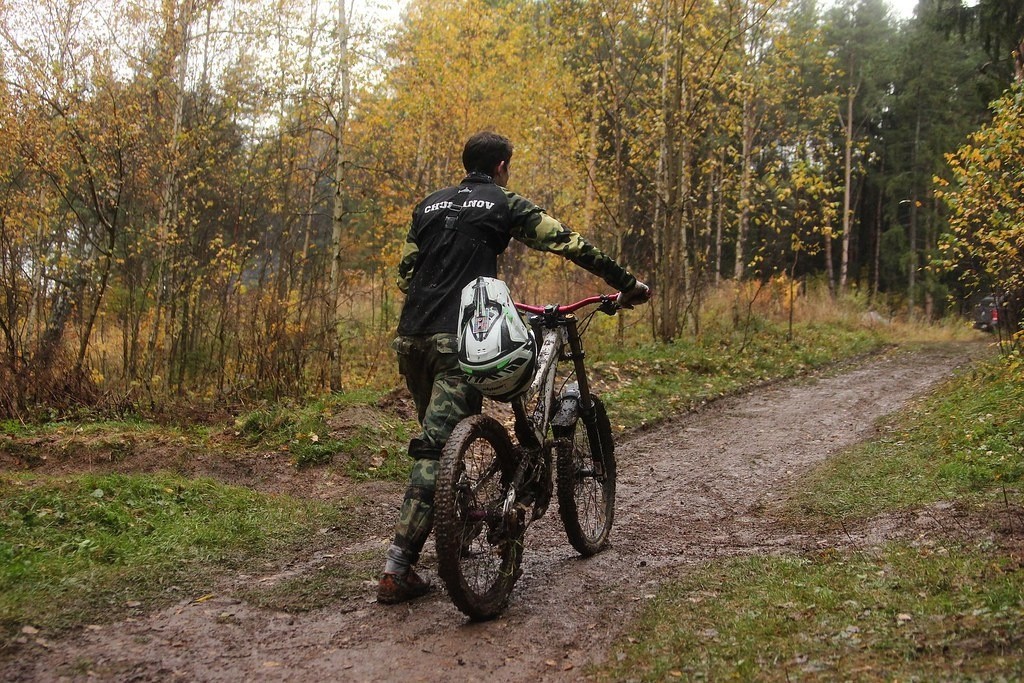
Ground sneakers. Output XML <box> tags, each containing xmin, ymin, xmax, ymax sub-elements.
<box><xmin>458</xmin><ymin>549</ymin><xmax>476</xmax><ymax>558</ymax></box>
<box><xmin>376</xmin><ymin>565</ymin><xmax>431</xmax><ymax>604</ymax></box>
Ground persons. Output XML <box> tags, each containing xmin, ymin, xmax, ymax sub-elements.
<box><xmin>376</xmin><ymin>133</ymin><xmax>651</xmax><ymax>605</ymax></box>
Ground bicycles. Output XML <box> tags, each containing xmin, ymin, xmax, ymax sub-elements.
<box><xmin>433</xmin><ymin>287</ymin><xmax>652</xmax><ymax>622</ymax></box>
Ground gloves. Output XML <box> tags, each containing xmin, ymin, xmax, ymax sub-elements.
<box><xmin>616</xmin><ymin>279</ymin><xmax>649</xmax><ymax>309</ymax></box>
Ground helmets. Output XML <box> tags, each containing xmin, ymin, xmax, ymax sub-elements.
<box><xmin>455</xmin><ymin>276</ymin><xmax>539</xmax><ymax>403</ymax></box>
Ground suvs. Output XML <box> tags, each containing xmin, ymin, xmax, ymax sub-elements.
<box><xmin>973</xmin><ymin>291</ymin><xmax>1024</xmax><ymax>334</ymax></box>
